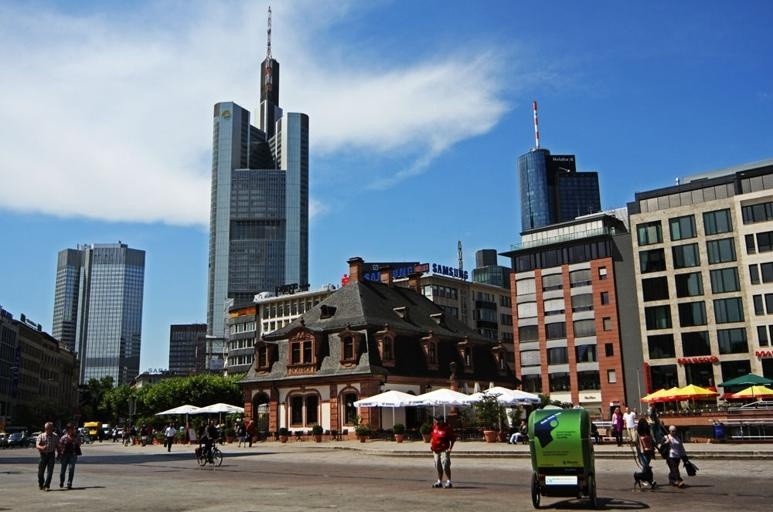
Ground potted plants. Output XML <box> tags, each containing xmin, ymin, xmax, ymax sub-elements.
<box><xmin>311</xmin><ymin>424</ymin><xmax>324</xmax><ymax>444</ymax></box>
<box><xmin>392</xmin><ymin>422</ymin><xmax>406</xmax><ymax>444</ymax></box>
<box><xmin>355</xmin><ymin>425</ymin><xmax>370</xmax><ymax>444</ymax></box>
<box><xmin>278</xmin><ymin>427</ymin><xmax>290</xmax><ymax>444</ymax></box>
<box><xmin>419</xmin><ymin>422</ymin><xmax>434</xmax><ymax>444</ymax></box>
<box><xmin>472</xmin><ymin>393</ymin><xmax>501</xmax><ymax>443</ymax></box>
<box><xmin>131</xmin><ymin>422</ymin><xmax>261</xmax><ymax>447</ymax></box>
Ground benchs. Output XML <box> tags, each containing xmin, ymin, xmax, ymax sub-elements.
<box><xmin>723</xmin><ymin>417</ymin><xmax>773</xmax><ymax>441</ymax></box>
<box><xmin>591</xmin><ymin>420</ymin><xmax>657</xmax><ymax>441</ymax></box>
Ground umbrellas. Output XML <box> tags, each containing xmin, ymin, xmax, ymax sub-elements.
<box><xmin>188</xmin><ymin>402</ymin><xmax>243</xmax><ymax>428</ymax></box>
<box><xmin>717</xmin><ymin>374</ymin><xmax>772</xmax><ymax>401</ymax></box>
<box><xmin>640</xmin><ymin>384</ymin><xmax>773</xmax><ymax>411</ymax></box>
<box><xmin>462</xmin><ymin>386</ymin><xmax>541</xmax><ymax>430</ymax></box>
<box><xmin>152</xmin><ymin>404</ymin><xmax>200</xmax><ymax>429</ymax></box>
<box><xmin>398</xmin><ymin>388</ymin><xmax>469</xmax><ymax>423</ymax></box>
<box><xmin>352</xmin><ymin>390</ymin><xmax>416</xmax><ymax>427</ymax></box>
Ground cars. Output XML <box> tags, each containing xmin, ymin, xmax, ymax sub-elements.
<box><xmin>0</xmin><ymin>429</ymin><xmax>57</xmax><ymax>447</ymax></box>
<box><xmin>726</xmin><ymin>401</ymin><xmax>772</xmax><ymax>414</ymax></box>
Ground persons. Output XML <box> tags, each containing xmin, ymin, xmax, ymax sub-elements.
<box><xmin>610</xmin><ymin>406</ymin><xmax>687</xmax><ymax>489</ymax></box>
<box><xmin>429</xmin><ymin>412</ymin><xmax>455</xmax><ymax>489</ymax></box>
<box><xmin>200</xmin><ymin>419</ymin><xmax>217</xmax><ymax>464</ymax></box>
<box><xmin>508</xmin><ymin>419</ymin><xmax>527</xmax><ymax>444</ymax></box>
<box><xmin>0</xmin><ymin>420</ymin><xmax>256</xmax><ymax>452</ymax></box>
<box><xmin>35</xmin><ymin>422</ymin><xmax>60</xmax><ymax>491</ymax></box>
<box><xmin>56</xmin><ymin>422</ymin><xmax>80</xmax><ymax>489</ymax></box>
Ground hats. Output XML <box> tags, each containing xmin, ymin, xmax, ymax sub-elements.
<box><xmin>433</xmin><ymin>415</ymin><xmax>444</xmax><ymax>421</ymax></box>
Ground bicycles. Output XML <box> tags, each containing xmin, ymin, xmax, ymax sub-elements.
<box><xmin>195</xmin><ymin>438</ymin><xmax>223</xmax><ymax>467</ymax></box>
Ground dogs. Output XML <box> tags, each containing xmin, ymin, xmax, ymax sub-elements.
<box><xmin>633</xmin><ymin>465</ymin><xmax>656</xmax><ymax>493</ymax></box>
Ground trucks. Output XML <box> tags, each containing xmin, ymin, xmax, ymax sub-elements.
<box><xmin>76</xmin><ymin>421</ymin><xmax>123</xmax><ymax>443</ymax></box>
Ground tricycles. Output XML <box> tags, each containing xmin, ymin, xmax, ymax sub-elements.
<box><xmin>526</xmin><ymin>408</ymin><xmax>600</xmax><ymax>509</ymax></box>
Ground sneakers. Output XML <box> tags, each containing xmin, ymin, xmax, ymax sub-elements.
<box><xmin>445</xmin><ymin>482</ymin><xmax>453</xmax><ymax>488</ymax></box>
<box><xmin>432</xmin><ymin>482</ymin><xmax>443</xmax><ymax>488</ymax></box>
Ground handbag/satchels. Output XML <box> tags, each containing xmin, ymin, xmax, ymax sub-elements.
<box><xmin>640</xmin><ymin>435</ymin><xmax>655</xmax><ymax>452</ymax></box>
<box><xmin>685</xmin><ymin>463</ymin><xmax>697</xmax><ymax>476</ymax></box>
<box><xmin>659</xmin><ymin>443</ymin><xmax>669</xmax><ymax>459</ymax></box>
<box><xmin>75</xmin><ymin>443</ymin><xmax>82</xmax><ymax>456</ymax></box>
